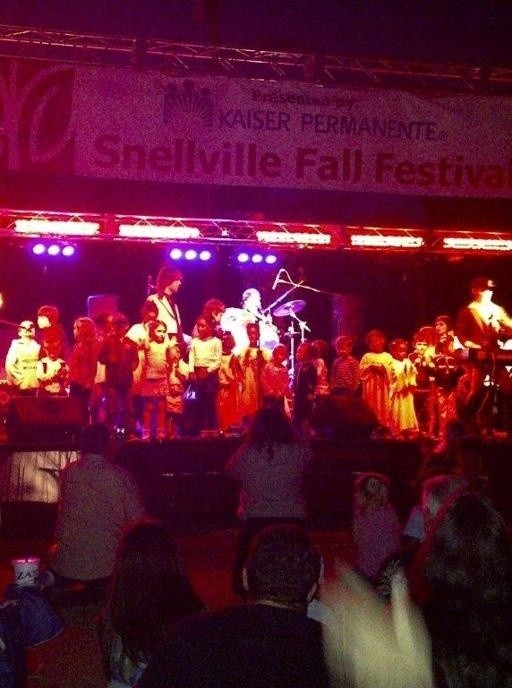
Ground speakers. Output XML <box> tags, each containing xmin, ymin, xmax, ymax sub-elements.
<box><xmin>8</xmin><ymin>395</ymin><xmax>83</xmax><ymax>445</ymax></box>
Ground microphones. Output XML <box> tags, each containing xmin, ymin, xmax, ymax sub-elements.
<box><xmin>272</xmin><ymin>268</ymin><xmax>285</xmax><ymax>291</ymax></box>
<box><xmin>246</xmin><ymin>305</ymin><xmax>269</xmax><ymax>325</ymax></box>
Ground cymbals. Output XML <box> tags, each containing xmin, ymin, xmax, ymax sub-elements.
<box><xmin>273</xmin><ymin>299</ymin><xmax>306</xmax><ymax>316</ymax></box>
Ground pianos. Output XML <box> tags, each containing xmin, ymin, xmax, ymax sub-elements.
<box><xmin>457</xmin><ymin>345</ymin><xmax>511</xmax><ymax>365</ymax></box>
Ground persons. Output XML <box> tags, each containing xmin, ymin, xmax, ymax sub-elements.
<box><xmin>0</xmin><ymin>262</ymin><xmax>512</xmax><ymax>688</ymax></box>
